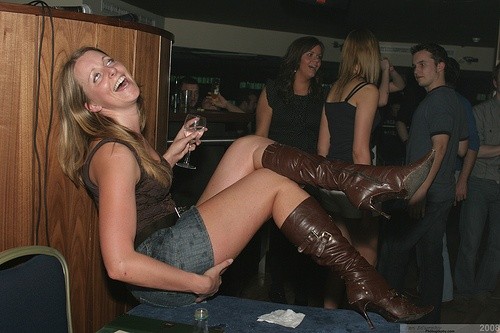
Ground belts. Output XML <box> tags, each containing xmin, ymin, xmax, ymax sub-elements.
<box><xmin>134</xmin><ymin>206</ymin><xmax>187</xmax><ymax>251</ymax></box>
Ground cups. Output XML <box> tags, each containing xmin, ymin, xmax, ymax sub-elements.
<box><xmin>180</xmin><ymin>90</ymin><xmax>192</xmax><ymax>112</ymax></box>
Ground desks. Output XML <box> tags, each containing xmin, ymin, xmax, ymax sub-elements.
<box><xmin>97</xmin><ymin>295</ymin><xmax>415</xmax><ymax>333</ymax></box>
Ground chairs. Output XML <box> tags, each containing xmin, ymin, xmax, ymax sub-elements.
<box><xmin>0</xmin><ymin>246</ymin><xmax>73</xmax><ymax>333</ymax></box>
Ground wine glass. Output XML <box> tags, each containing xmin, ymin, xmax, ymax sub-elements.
<box><xmin>175</xmin><ymin>114</ymin><xmax>207</xmax><ymax>169</ymax></box>
<box><xmin>210</xmin><ymin>78</ymin><xmax>222</xmax><ymax>110</ymax></box>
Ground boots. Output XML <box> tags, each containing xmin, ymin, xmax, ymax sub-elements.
<box><xmin>281</xmin><ymin>196</ymin><xmax>441</xmax><ymax>329</ymax></box>
<box><xmin>262</xmin><ymin>143</ymin><xmax>435</xmax><ymax>219</ymax></box>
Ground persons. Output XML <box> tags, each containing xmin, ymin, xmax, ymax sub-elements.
<box><xmin>177</xmin><ymin>77</ymin><xmax>199</xmax><ymax>109</ymax></box>
<box><xmin>59</xmin><ymin>46</ymin><xmax>437</xmax><ymax>329</ymax></box>
<box><xmin>370</xmin><ymin>41</ymin><xmax>480</xmax><ymax>324</ymax></box>
<box><xmin>451</xmin><ymin>64</ymin><xmax>499</xmax><ymax>298</ymax></box>
<box><xmin>255</xmin><ymin>36</ymin><xmax>327</xmax><ymax>306</ymax></box>
<box><xmin>316</xmin><ymin>28</ymin><xmax>382</xmax><ymax>311</ymax></box>
<box><xmin>202</xmin><ymin>85</ymin><xmax>256</xmax><ymax>115</ymax></box>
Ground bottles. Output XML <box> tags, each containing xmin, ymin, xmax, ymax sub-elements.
<box><xmin>203</xmin><ymin>92</ymin><xmax>212</xmax><ymax>110</ymax></box>
<box><xmin>192</xmin><ymin>308</ymin><xmax>209</xmax><ymax>333</ymax></box>
